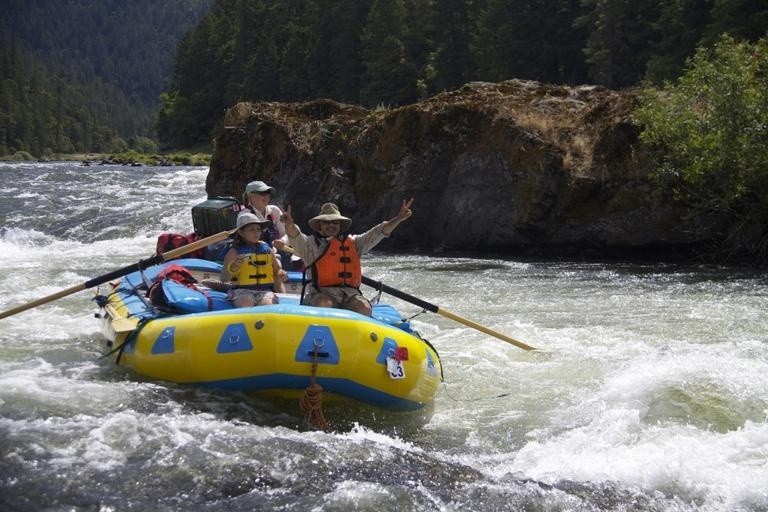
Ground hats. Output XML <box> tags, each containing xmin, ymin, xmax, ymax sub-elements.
<box><xmin>308</xmin><ymin>203</ymin><xmax>353</xmax><ymax>233</ymax></box>
<box><xmin>228</xmin><ymin>213</ymin><xmax>271</xmax><ymax>240</ymax></box>
<box><xmin>245</xmin><ymin>180</ymin><xmax>276</xmax><ymax>195</ymax></box>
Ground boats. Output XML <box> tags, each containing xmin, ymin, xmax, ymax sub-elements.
<box><xmin>100</xmin><ymin>253</ymin><xmax>441</xmax><ymax>416</ymax></box>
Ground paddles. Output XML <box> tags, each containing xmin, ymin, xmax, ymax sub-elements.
<box><xmin>105</xmin><ymin>304</ymin><xmax>138</xmax><ymax>334</ymax></box>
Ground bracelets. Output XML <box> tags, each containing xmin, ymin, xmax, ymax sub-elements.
<box><xmin>227</xmin><ymin>261</ymin><xmax>242</xmax><ymax>275</ymax></box>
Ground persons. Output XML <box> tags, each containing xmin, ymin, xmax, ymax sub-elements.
<box><xmin>220</xmin><ymin>213</ymin><xmax>288</xmax><ymax>308</ymax></box>
<box><xmin>279</xmin><ymin>197</ymin><xmax>415</xmax><ymax>318</ymax></box>
<box><xmin>237</xmin><ymin>181</ymin><xmax>293</xmax><ymax>272</ymax></box>
